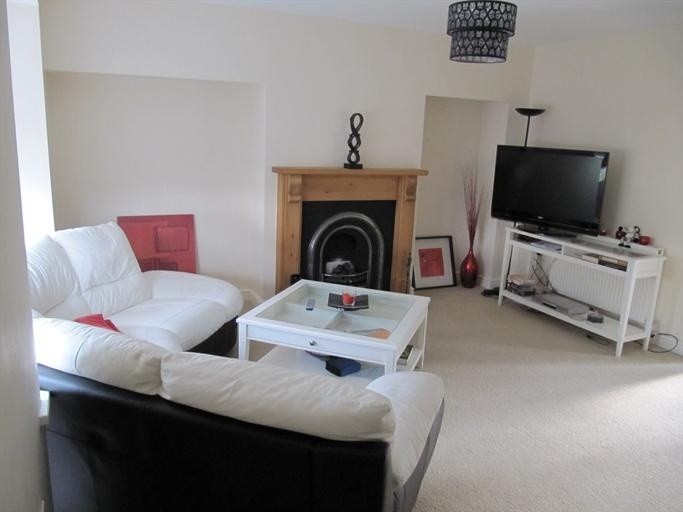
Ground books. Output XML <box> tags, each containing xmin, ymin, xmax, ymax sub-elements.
<box><xmin>398</xmin><ymin>345</ymin><xmax>413</xmax><ymax>365</ymax></box>
<box><xmin>582</xmin><ymin>254</ymin><xmax>628</xmax><ymax>272</ymax></box>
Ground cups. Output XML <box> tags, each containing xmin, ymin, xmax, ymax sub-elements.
<box><xmin>343</xmin><ymin>286</ymin><xmax>357</xmax><ymax>307</ymax></box>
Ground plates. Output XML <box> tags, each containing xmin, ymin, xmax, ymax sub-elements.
<box><xmin>328</xmin><ymin>293</ymin><xmax>369</xmax><ymax>312</ymax></box>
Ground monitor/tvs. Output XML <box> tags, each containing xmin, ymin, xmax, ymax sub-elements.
<box><xmin>491</xmin><ymin>144</ymin><xmax>610</xmax><ymax>238</ymax></box>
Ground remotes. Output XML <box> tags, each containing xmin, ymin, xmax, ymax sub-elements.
<box><xmin>305</xmin><ymin>297</ymin><xmax>316</xmax><ymax>310</ymax></box>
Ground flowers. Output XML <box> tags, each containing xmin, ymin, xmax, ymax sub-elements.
<box><xmin>460</xmin><ymin>167</ymin><xmax>483</xmax><ymax>249</ymax></box>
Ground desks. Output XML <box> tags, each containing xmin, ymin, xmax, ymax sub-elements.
<box><xmin>497</xmin><ymin>227</ymin><xmax>667</xmax><ymax>356</ymax></box>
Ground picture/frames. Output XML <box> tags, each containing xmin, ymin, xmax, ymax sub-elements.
<box><xmin>411</xmin><ymin>235</ymin><xmax>457</xmax><ymax>289</ymax></box>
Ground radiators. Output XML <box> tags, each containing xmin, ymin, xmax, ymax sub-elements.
<box><xmin>547</xmin><ymin>257</ymin><xmax>652</xmax><ymax>324</ymax></box>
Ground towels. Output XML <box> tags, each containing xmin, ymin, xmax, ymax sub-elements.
<box><xmin>72</xmin><ymin>313</ymin><xmax>119</xmax><ymax>332</ymax></box>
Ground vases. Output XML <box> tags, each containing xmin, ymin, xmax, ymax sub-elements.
<box><xmin>460</xmin><ymin>246</ymin><xmax>477</xmax><ymax>287</ymax></box>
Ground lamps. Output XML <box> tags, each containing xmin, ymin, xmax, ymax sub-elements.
<box><xmin>446</xmin><ymin>1</ymin><xmax>517</xmax><ymax>63</ymax></box>
<box><xmin>481</xmin><ymin>108</ymin><xmax>546</xmax><ymax>296</ymax></box>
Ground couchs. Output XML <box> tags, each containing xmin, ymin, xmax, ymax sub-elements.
<box><xmin>26</xmin><ymin>222</ymin><xmax>446</xmax><ymax>510</ymax></box>
